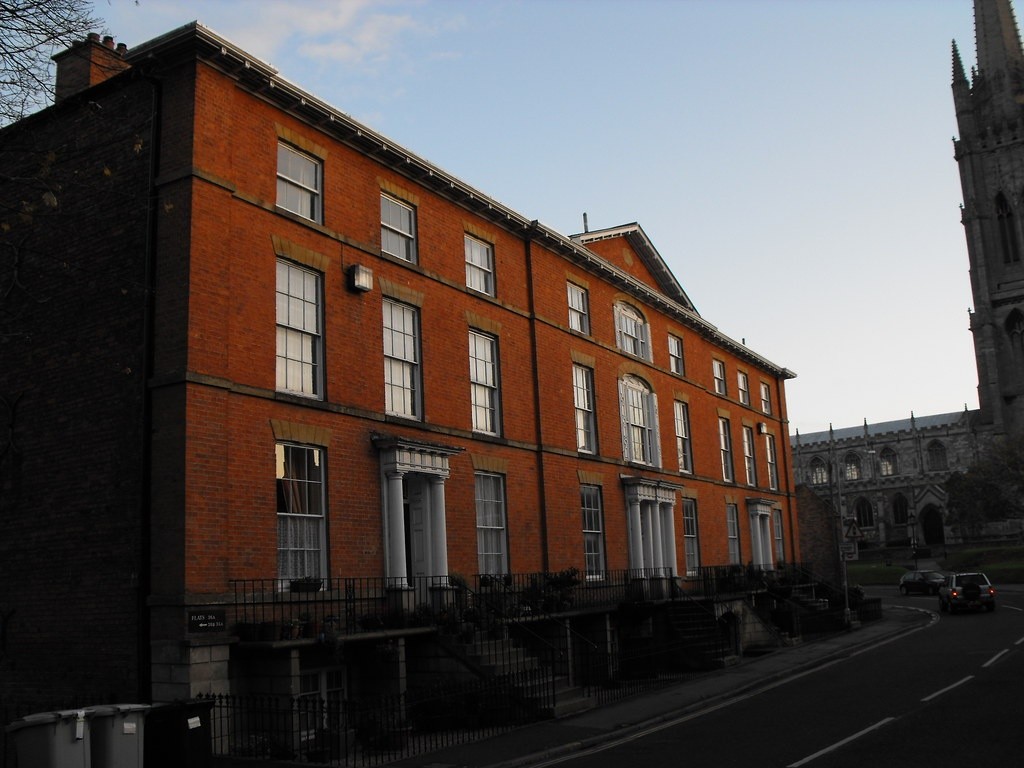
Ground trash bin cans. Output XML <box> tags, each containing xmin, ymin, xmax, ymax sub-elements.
<box><xmin>81</xmin><ymin>703</ymin><xmax>153</xmax><ymax>768</ymax></box>
<box><xmin>5</xmin><ymin>709</ymin><xmax>96</xmax><ymax>768</ymax></box>
<box><xmin>144</xmin><ymin>694</ymin><xmax>216</xmax><ymax>768</ymax></box>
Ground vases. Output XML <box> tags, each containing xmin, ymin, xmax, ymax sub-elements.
<box><xmin>307</xmin><ymin>725</ymin><xmax>355</xmax><ymax>765</ymax></box>
<box><xmin>236</xmin><ymin>622</ymin><xmax>281</xmax><ymax>640</ymax></box>
<box><xmin>381</xmin><ymin>725</ymin><xmax>412</xmax><ymax>751</ymax></box>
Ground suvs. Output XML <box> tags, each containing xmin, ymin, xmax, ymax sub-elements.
<box><xmin>937</xmin><ymin>573</ymin><xmax>998</xmax><ymax>615</ymax></box>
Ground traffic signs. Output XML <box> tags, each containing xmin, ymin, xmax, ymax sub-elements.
<box><xmin>187</xmin><ymin>609</ymin><xmax>227</xmax><ymax>634</ymax></box>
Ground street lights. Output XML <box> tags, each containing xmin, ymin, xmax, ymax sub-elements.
<box><xmin>908</xmin><ymin>512</ymin><xmax>919</xmax><ymax>570</ymax></box>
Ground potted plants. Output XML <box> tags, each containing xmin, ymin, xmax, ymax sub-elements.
<box><xmin>284</xmin><ymin>613</ymin><xmax>321</xmax><ymax>639</ymax></box>
<box><xmin>290</xmin><ymin>575</ymin><xmax>322</xmax><ymax>592</ymax></box>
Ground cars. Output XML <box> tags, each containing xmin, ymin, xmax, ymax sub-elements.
<box><xmin>899</xmin><ymin>570</ymin><xmax>947</xmax><ymax>596</ymax></box>
